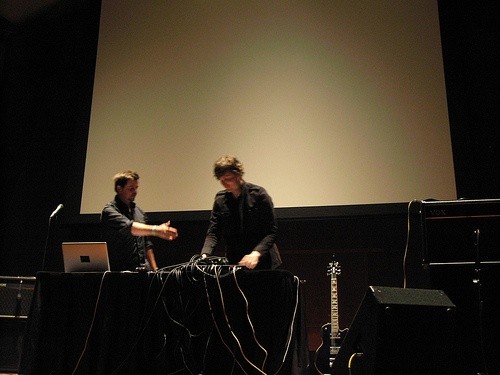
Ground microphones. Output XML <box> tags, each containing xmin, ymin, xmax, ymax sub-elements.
<box><xmin>50</xmin><ymin>204</ymin><xmax>64</xmax><ymax>218</ymax></box>
<box><xmin>17</xmin><ymin>280</ymin><xmax>22</xmax><ymax>300</ymax></box>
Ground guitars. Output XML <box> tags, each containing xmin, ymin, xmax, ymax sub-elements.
<box><xmin>315</xmin><ymin>258</ymin><xmax>348</xmax><ymax>375</ymax></box>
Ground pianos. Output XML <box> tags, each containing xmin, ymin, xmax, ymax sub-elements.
<box><xmin>403</xmin><ymin>200</ymin><xmax>500</xmax><ymax>291</ymax></box>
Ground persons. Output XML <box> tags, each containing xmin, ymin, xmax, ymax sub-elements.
<box><xmin>99</xmin><ymin>169</ymin><xmax>178</xmax><ymax>271</ymax></box>
<box><xmin>199</xmin><ymin>155</ymin><xmax>284</xmax><ymax>270</ymax></box>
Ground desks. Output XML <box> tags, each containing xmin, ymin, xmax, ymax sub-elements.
<box><xmin>18</xmin><ymin>270</ymin><xmax>306</xmax><ymax>375</ymax></box>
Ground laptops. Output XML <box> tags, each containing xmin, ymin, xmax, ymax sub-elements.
<box><xmin>61</xmin><ymin>241</ymin><xmax>111</xmax><ymax>272</ymax></box>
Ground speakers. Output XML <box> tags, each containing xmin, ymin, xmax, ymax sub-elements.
<box><xmin>0</xmin><ymin>276</ymin><xmax>36</xmax><ymax>315</ymax></box>
<box><xmin>419</xmin><ymin>198</ymin><xmax>500</xmax><ymax>265</ymax></box>
<box><xmin>333</xmin><ymin>285</ymin><xmax>459</xmax><ymax>375</ymax></box>
